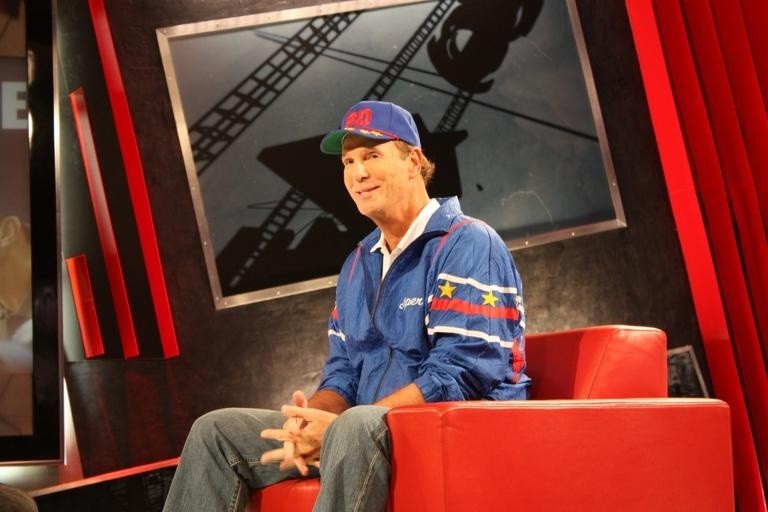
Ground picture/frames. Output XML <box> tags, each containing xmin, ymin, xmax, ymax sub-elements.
<box><xmin>154</xmin><ymin>1</ymin><xmax>627</xmax><ymax>312</ymax></box>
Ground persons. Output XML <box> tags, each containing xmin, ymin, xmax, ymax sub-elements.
<box><xmin>159</xmin><ymin>96</ymin><xmax>539</xmax><ymax>512</ymax></box>
<box><xmin>1</xmin><ymin>128</ymin><xmax>36</xmax><ymax>327</ymax></box>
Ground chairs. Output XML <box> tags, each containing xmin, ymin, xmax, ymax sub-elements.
<box><xmin>247</xmin><ymin>324</ymin><xmax>736</xmax><ymax>512</ymax></box>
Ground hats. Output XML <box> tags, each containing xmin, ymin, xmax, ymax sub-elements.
<box><xmin>320</xmin><ymin>100</ymin><xmax>422</xmax><ymax>154</ymax></box>
